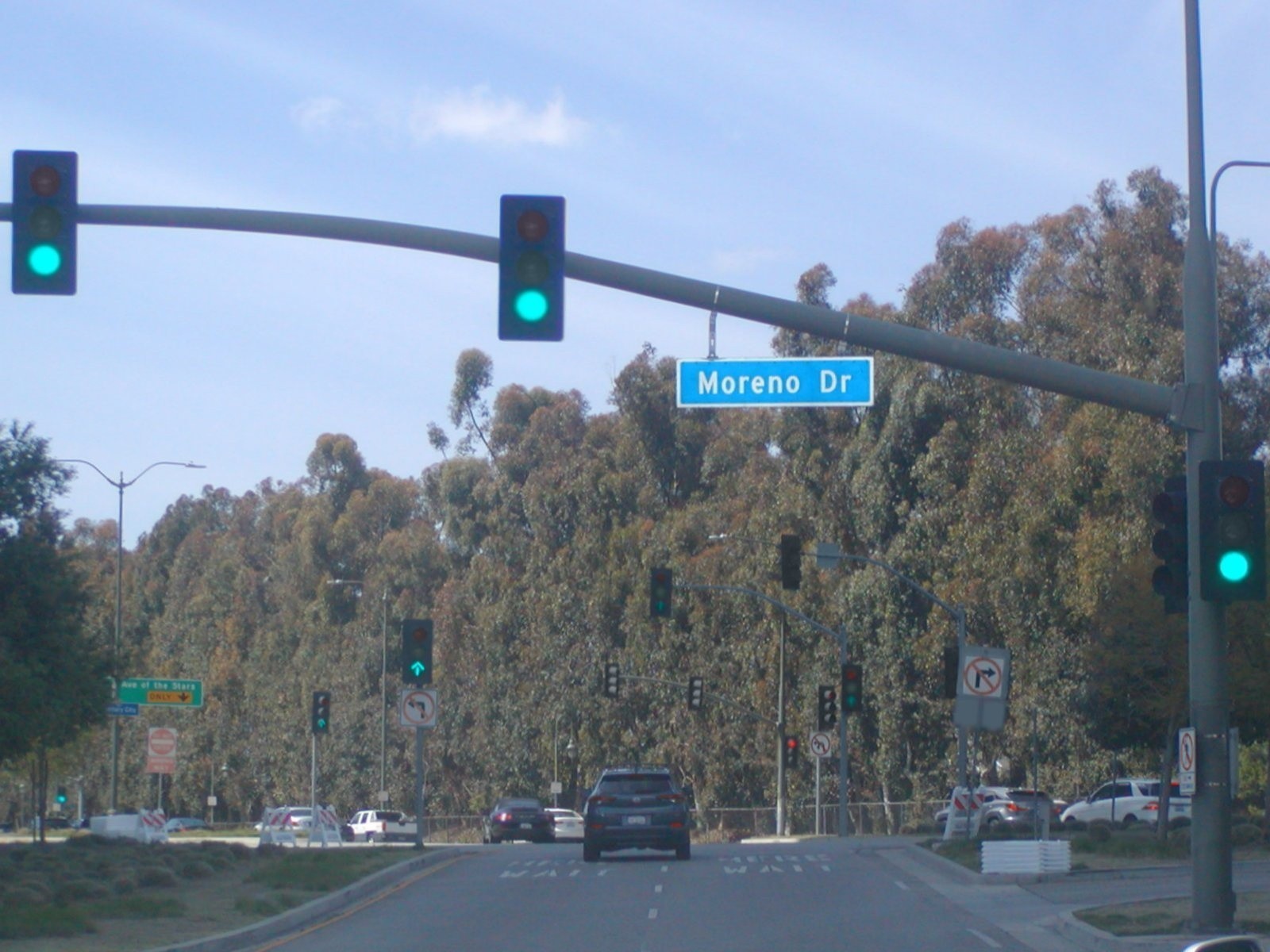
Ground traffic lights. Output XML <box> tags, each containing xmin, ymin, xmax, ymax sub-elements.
<box><xmin>780</xmin><ymin>534</ymin><xmax>803</xmax><ymax>590</ymax></box>
<box><xmin>1199</xmin><ymin>459</ymin><xmax>1270</xmax><ymax>605</ymax></box>
<box><xmin>785</xmin><ymin>736</ymin><xmax>799</xmax><ymax>769</ymax></box>
<box><xmin>1151</xmin><ymin>475</ymin><xmax>1189</xmax><ymax>615</ymax></box>
<box><xmin>649</xmin><ymin>567</ymin><xmax>673</xmax><ymax>618</ymax></box>
<box><xmin>604</xmin><ymin>662</ymin><xmax>620</xmax><ymax>698</ymax></box>
<box><xmin>11</xmin><ymin>150</ymin><xmax>77</xmax><ymax>296</ymax></box>
<box><xmin>311</xmin><ymin>692</ymin><xmax>329</xmax><ymax>734</ymax></box>
<box><xmin>817</xmin><ymin>684</ymin><xmax>838</xmax><ymax>732</ymax></box>
<box><xmin>689</xmin><ymin>676</ymin><xmax>702</xmax><ymax>710</ymax></box>
<box><xmin>402</xmin><ymin>618</ymin><xmax>433</xmax><ymax>685</ymax></box>
<box><xmin>842</xmin><ymin>664</ymin><xmax>862</xmax><ymax>713</ymax></box>
<box><xmin>498</xmin><ymin>194</ymin><xmax>566</xmax><ymax>342</ymax></box>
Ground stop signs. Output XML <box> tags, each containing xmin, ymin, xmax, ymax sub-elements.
<box><xmin>147</xmin><ymin>727</ymin><xmax>177</xmax><ymax>758</ymax></box>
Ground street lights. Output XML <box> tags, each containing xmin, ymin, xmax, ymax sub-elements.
<box><xmin>52</xmin><ymin>459</ymin><xmax>208</xmax><ymax>815</ymax></box>
<box><xmin>710</xmin><ymin>533</ymin><xmax>787</xmax><ymax>836</ymax></box>
<box><xmin>325</xmin><ymin>579</ymin><xmax>388</xmax><ymax>810</ymax></box>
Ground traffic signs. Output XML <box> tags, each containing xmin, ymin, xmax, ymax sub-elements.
<box><xmin>55</xmin><ymin>785</ymin><xmax>67</xmax><ymax>804</ymax></box>
<box><xmin>105</xmin><ymin>704</ymin><xmax>139</xmax><ymax>716</ymax></box>
<box><xmin>117</xmin><ymin>677</ymin><xmax>204</xmax><ymax>708</ymax></box>
<box><xmin>400</xmin><ymin>689</ymin><xmax>437</xmax><ymax>728</ymax></box>
<box><xmin>146</xmin><ymin>756</ymin><xmax>174</xmax><ymax>773</ymax></box>
<box><xmin>677</xmin><ymin>357</ymin><xmax>874</xmax><ymax>407</ymax></box>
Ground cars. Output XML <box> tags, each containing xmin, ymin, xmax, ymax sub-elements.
<box><xmin>254</xmin><ymin>806</ymin><xmax>417</xmax><ymax>844</ymax></box>
<box><xmin>28</xmin><ymin>815</ymin><xmax>214</xmax><ymax>834</ymax></box>
<box><xmin>482</xmin><ymin>796</ymin><xmax>585</xmax><ymax>844</ymax></box>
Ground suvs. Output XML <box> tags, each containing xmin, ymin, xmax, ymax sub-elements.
<box><xmin>1059</xmin><ymin>777</ymin><xmax>1191</xmax><ymax>831</ymax></box>
<box><xmin>936</xmin><ymin>787</ymin><xmax>1058</xmax><ymax>834</ymax></box>
<box><xmin>585</xmin><ymin>765</ymin><xmax>690</xmax><ymax>860</ymax></box>
